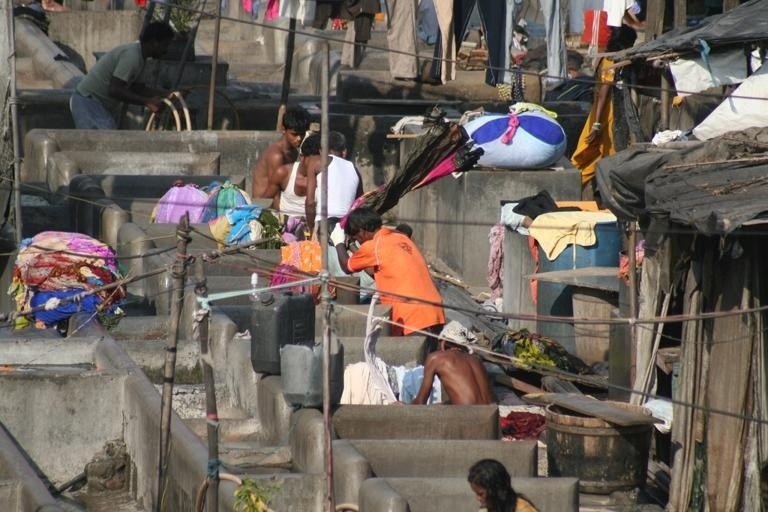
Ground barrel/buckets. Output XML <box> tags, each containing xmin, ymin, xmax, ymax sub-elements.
<box><xmin>572</xmin><ymin>293</ymin><xmax>620</xmax><ymax>365</ymax></box>
<box><xmin>546</xmin><ymin>400</ymin><xmax>651</xmax><ymax>496</ymax></box>
<box><xmin>336</xmin><ymin>277</ymin><xmax>360</xmax><ymax>305</ymax></box>
<box><xmin>536</xmin><ymin>222</ymin><xmax>621</xmax><ymax>356</ymax></box>
<box><xmin>279</xmin><ymin>337</ymin><xmax>344</xmax><ymax>409</ymax></box>
<box><xmin>251</xmin><ymin>290</ymin><xmax>315</xmax><ymax>374</ymax></box>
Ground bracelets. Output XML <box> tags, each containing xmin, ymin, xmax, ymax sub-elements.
<box><xmin>592</xmin><ymin>122</ymin><xmax>602</xmax><ymax>131</ymax></box>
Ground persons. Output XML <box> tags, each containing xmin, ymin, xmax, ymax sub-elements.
<box><xmin>304</xmin><ymin>129</ymin><xmax>365</xmax><ymax>245</ymax></box>
<box><xmin>250</xmin><ymin>104</ymin><xmax>311</xmax><ymax>213</ymax></box>
<box><xmin>467</xmin><ymin>457</ymin><xmax>538</xmax><ymax>511</ymax></box>
<box><xmin>329</xmin><ymin>201</ymin><xmax>447</xmax><ymax>359</ymax></box>
<box><xmin>402</xmin><ymin>317</ymin><xmax>497</xmax><ymax>408</ymax></box>
<box><xmin>263</xmin><ymin>132</ymin><xmax>322</xmax><ymax>223</ymax></box>
<box><xmin>572</xmin><ymin>23</ymin><xmax>647</xmax><ymax>192</ymax></box>
<box><xmin>67</xmin><ymin>19</ymin><xmax>176</xmax><ymax>132</ymax></box>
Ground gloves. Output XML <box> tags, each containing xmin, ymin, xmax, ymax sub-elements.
<box><xmin>330</xmin><ymin>221</ymin><xmax>346</xmax><ymax>247</ymax></box>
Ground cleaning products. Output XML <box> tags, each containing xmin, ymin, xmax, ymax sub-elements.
<box><xmin>250</xmin><ymin>272</ymin><xmax>261</xmax><ymax>301</ymax></box>
